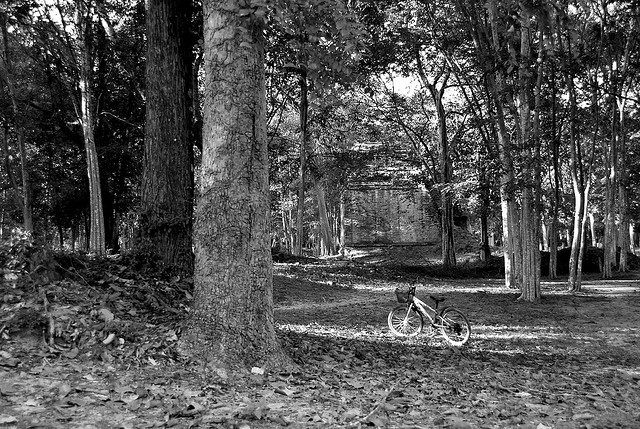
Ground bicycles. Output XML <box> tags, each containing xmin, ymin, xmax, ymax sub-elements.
<box><xmin>387</xmin><ymin>275</ymin><xmax>472</xmax><ymax>347</ymax></box>
